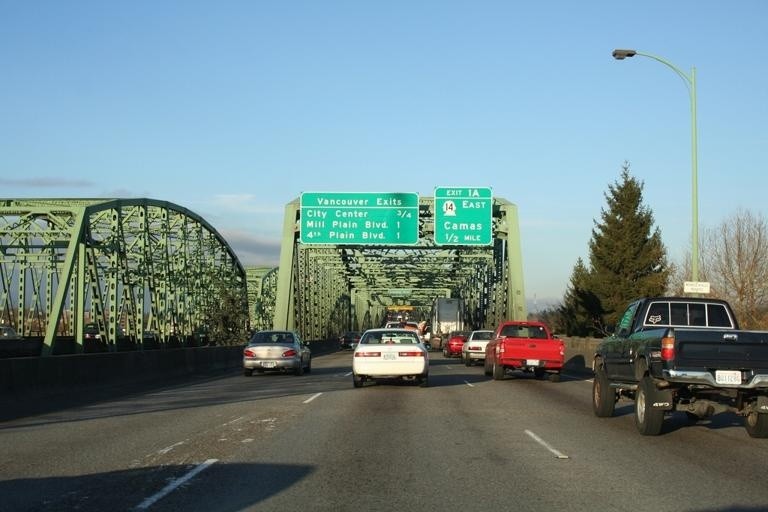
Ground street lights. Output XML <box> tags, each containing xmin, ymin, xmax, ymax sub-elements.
<box><xmin>609</xmin><ymin>49</ymin><xmax>708</xmax><ymax>292</ymax></box>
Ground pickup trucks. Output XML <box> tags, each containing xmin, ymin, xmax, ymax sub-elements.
<box><xmin>590</xmin><ymin>297</ymin><xmax>767</xmax><ymax>437</ymax></box>
<box><xmin>483</xmin><ymin>321</ymin><xmax>565</xmax><ymax>382</ymax></box>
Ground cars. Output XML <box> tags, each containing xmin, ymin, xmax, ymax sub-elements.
<box><xmin>241</xmin><ymin>328</ymin><xmax>315</xmax><ymax>375</ymax></box>
<box><xmin>351</xmin><ymin>326</ymin><xmax>433</xmax><ymax>387</ymax></box>
<box><xmin>337</xmin><ymin>318</ymin><xmax>474</xmax><ymax>358</ymax></box>
<box><xmin>1</xmin><ymin>326</ymin><xmax>23</xmax><ymax>340</ymax></box>
<box><xmin>81</xmin><ymin>319</ymin><xmax>222</xmax><ymax>344</ymax></box>
<box><xmin>460</xmin><ymin>329</ymin><xmax>496</xmax><ymax>366</ymax></box>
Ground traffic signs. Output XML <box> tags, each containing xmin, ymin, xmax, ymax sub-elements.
<box><xmin>431</xmin><ymin>184</ymin><xmax>495</xmax><ymax>247</ymax></box>
<box><xmin>299</xmin><ymin>192</ymin><xmax>421</xmax><ymax>246</ymax></box>
<box><xmin>681</xmin><ymin>280</ymin><xmax>711</xmax><ymax>296</ymax></box>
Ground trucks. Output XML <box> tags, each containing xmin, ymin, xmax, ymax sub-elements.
<box><xmin>429</xmin><ymin>293</ymin><xmax>468</xmax><ymax>350</ymax></box>
